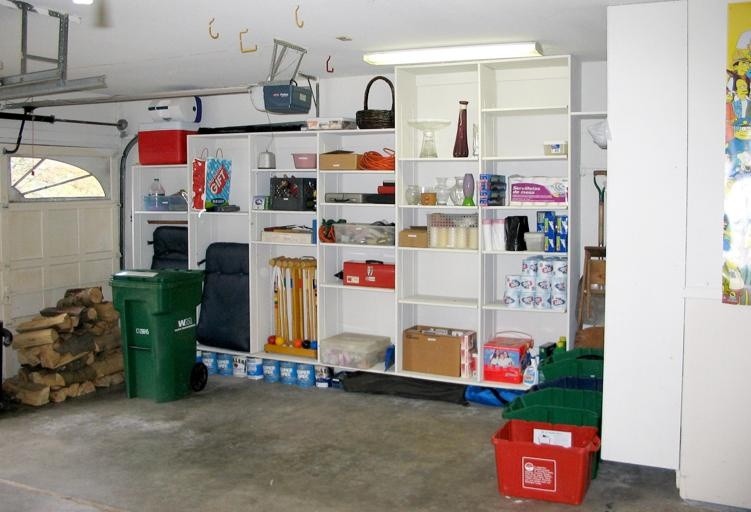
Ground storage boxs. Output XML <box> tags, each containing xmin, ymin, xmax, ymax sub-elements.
<box><xmin>483</xmin><ymin>338</ymin><xmax>534</xmax><ymax>384</ymax></box>
<box><xmin>491</xmin><ymin>348</ymin><xmax>604</xmax><ymax>505</ymax></box>
<box><xmin>403</xmin><ymin>325</ymin><xmax>472</xmax><ymax>377</ymax></box>
<box><xmin>138</xmin><ymin>120</ymin><xmax>200</xmax><ymax>165</ymax></box>
<box><xmin>264</xmin><ymin>85</ymin><xmax>311</xmax><ymax>113</ymax></box>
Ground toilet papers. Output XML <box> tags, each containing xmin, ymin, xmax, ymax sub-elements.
<box><xmin>503</xmin><ymin>258</ymin><xmax>568</xmax><ymax>309</ymax></box>
<box><xmin>430</xmin><ymin>225</ymin><xmax>478</xmax><ymax>250</ymax></box>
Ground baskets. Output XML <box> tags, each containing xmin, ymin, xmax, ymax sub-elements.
<box><xmin>357</xmin><ymin>75</ymin><xmax>394</xmax><ymax>127</ymax></box>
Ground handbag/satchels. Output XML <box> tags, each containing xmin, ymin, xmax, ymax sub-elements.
<box><xmin>192</xmin><ymin>148</ymin><xmax>231</xmax><ymax>210</ymax></box>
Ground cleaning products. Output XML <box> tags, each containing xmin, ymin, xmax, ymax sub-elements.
<box><xmin>524</xmin><ymin>348</ymin><xmax>539</xmax><ymax>386</ymax></box>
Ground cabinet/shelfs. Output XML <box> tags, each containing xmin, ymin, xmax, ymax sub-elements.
<box><xmin>394</xmin><ymin>54</ymin><xmax>581</xmax><ymax>392</ymax></box>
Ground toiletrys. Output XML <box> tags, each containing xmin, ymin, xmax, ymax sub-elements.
<box><xmin>544</xmin><ymin>216</ymin><xmax>568</xmax><ymax>252</ymax></box>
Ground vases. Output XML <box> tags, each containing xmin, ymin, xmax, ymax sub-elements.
<box><xmin>405</xmin><ymin>174</ymin><xmax>474</xmax><ymax>206</ymax></box>
<box><xmin>472</xmin><ymin>123</ymin><xmax>479</xmax><ymax>156</ymax></box>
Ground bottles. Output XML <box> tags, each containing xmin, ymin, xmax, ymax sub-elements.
<box><xmin>148</xmin><ymin>177</ymin><xmax>165</xmax><ymax>211</ymax></box>
<box><xmin>405</xmin><ymin>172</ymin><xmax>476</xmax><ymax>206</ymax></box>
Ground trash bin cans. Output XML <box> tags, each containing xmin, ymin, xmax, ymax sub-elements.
<box><xmin>108</xmin><ymin>268</ymin><xmax>208</xmax><ymax>404</ymax></box>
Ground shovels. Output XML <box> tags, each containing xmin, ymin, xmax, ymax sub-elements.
<box><xmin>574</xmin><ymin>171</ymin><xmax>606</xmax><ymax>326</ymax></box>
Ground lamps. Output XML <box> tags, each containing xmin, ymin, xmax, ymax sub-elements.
<box><xmin>363</xmin><ymin>41</ymin><xmax>544</xmax><ymax>66</ymax></box>
